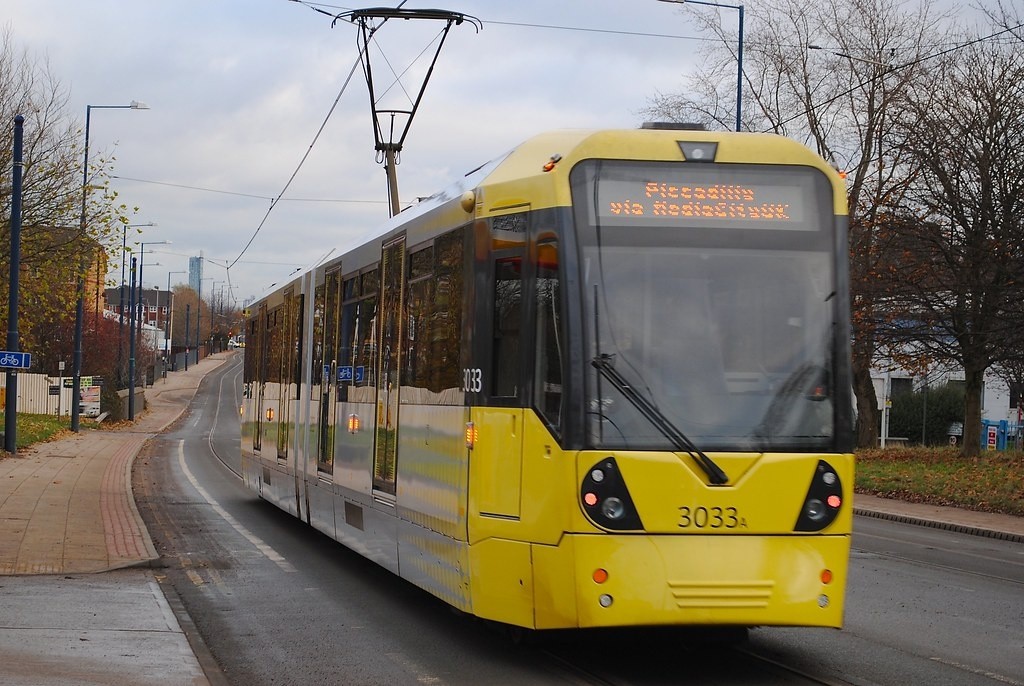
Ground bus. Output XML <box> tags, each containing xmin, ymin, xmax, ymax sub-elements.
<box><xmin>239</xmin><ymin>7</ymin><xmax>875</xmax><ymax>637</ymax></box>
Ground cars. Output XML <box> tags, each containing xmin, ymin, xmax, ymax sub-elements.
<box><xmin>228</xmin><ymin>340</ymin><xmax>239</xmax><ymax>349</ymax></box>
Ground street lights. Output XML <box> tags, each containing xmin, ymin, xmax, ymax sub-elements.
<box><xmin>134</xmin><ymin>240</ymin><xmax>173</xmax><ymax>354</ymax></box>
<box><xmin>163</xmin><ymin>271</ymin><xmax>188</xmax><ymax>380</ymax></box>
<box><xmin>195</xmin><ymin>277</ymin><xmax>214</xmax><ymax>364</ymax></box>
<box><xmin>70</xmin><ymin>100</ymin><xmax>151</xmax><ymax>434</ymax></box>
<box><xmin>115</xmin><ymin>222</ymin><xmax>160</xmax><ymax>385</ymax></box>
<box><xmin>126</xmin><ymin>250</ymin><xmax>155</xmax><ymax>332</ymax></box>
<box><xmin>152</xmin><ymin>284</ymin><xmax>160</xmax><ymax>352</ymax></box>
